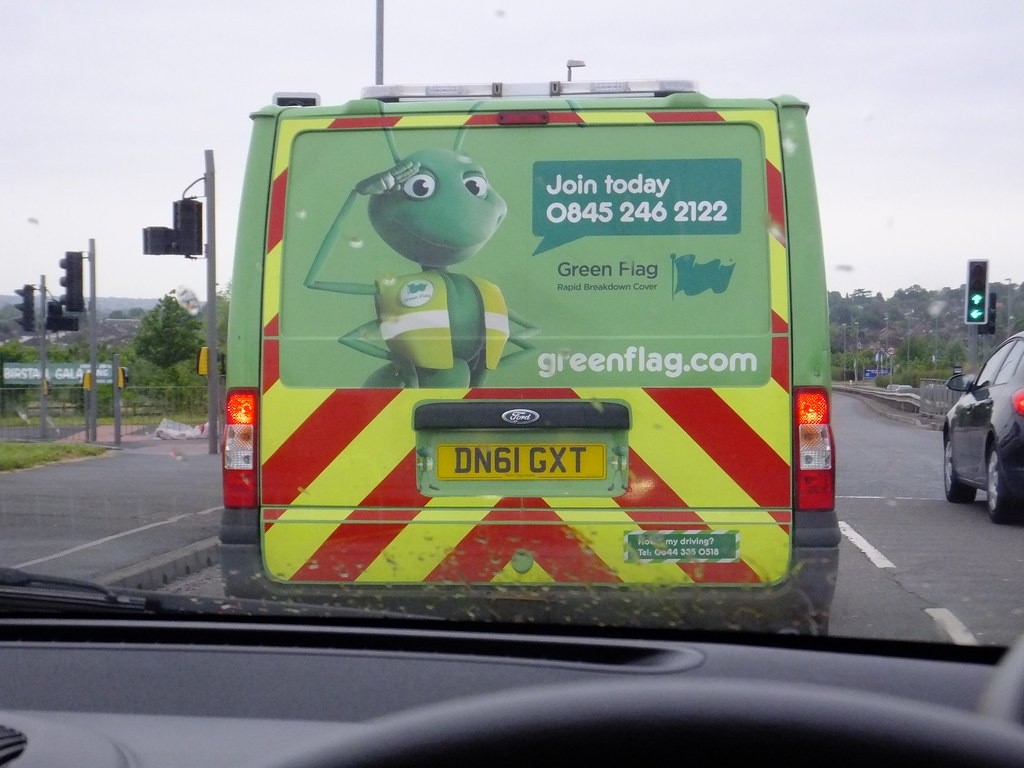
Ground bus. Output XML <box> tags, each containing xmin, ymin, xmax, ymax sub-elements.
<box><xmin>216</xmin><ymin>76</ymin><xmax>840</xmax><ymax>630</ymax></box>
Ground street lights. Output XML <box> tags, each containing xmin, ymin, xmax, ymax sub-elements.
<box><xmin>842</xmin><ymin>323</ymin><xmax>847</xmax><ymax>381</ymax></box>
<box><xmin>904</xmin><ymin>312</ymin><xmax>911</xmax><ymax>366</ymax></box>
<box><xmin>854</xmin><ymin>322</ymin><xmax>859</xmax><ymax>382</ymax></box>
<box><xmin>883</xmin><ymin>317</ymin><xmax>889</xmax><ymax>374</ymax></box>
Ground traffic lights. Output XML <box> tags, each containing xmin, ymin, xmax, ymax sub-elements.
<box><xmin>963</xmin><ymin>260</ymin><xmax>990</xmax><ymax>325</ymax></box>
<box><xmin>14</xmin><ymin>284</ymin><xmax>34</xmax><ymax>330</ymax></box>
<box><xmin>46</xmin><ymin>301</ymin><xmax>78</xmax><ymax>332</ymax></box>
<box><xmin>60</xmin><ymin>252</ymin><xmax>86</xmax><ymax>312</ymax></box>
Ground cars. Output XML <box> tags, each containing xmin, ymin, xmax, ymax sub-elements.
<box><xmin>942</xmin><ymin>330</ymin><xmax>1024</xmax><ymax>525</ymax></box>
